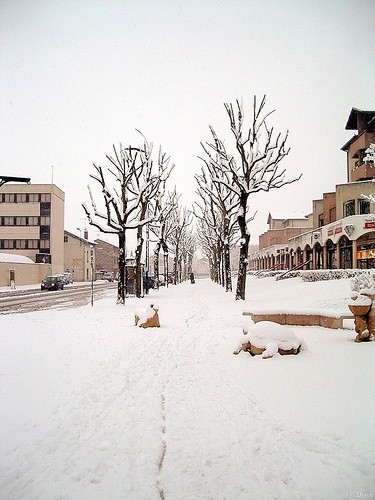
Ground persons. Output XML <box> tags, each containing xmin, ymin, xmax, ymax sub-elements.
<box><xmin>190</xmin><ymin>273</ymin><xmax>194</xmax><ymax>284</ymax></box>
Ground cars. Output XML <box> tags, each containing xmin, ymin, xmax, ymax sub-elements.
<box><xmin>101</xmin><ymin>273</ymin><xmax>114</xmax><ymax>282</ymax></box>
<box><xmin>40</xmin><ymin>275</ymin><xmax>64</xmax><ymax>291</ymax></box>
<box><xmin>143</xmin><ymin>272</ymin><xmax>176</xmax><ymax>289</ymax></box>
<box><xmin>55</xmin><ymin>272</ymin><xmax>74</xmax><ymax>285</ymax></box>
